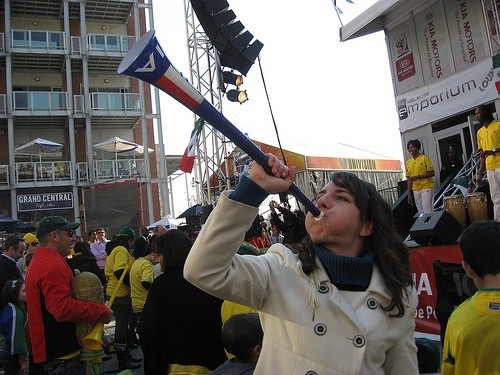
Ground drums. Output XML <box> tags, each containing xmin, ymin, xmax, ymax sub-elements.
<box><xmin>444</xmin><ymin>194</ymin><xmax>466</xmax><ymax>228</ymax></box>
<box><xmin>466</xmin><ymin>192</ymin><xmax>488</xmax><ymax>224</ymax></box>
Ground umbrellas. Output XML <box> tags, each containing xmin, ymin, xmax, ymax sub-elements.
<box><xmin>16</xmin><ymin>138</ymin><xmax>63</xmax><ymax>178</ymax></box>
<box><xmin>93</xmin><ymin>137</ymin><xmax>154</xmax><ymax>176</ymax></box>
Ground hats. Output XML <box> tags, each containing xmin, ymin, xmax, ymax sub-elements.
<box><xmin>22</xmin><ymin>233</ymin><xmax>38</xmax><ymax>244</ymax></box>
<box><xmin>36</xmin><ymin>216</ymin><xmax>80</xmax><ymax>239</ymax></box>
<box><xmin>119</xmin><ymin>226</ymin><xmax>136</xmax><ymax>238</ymax></box>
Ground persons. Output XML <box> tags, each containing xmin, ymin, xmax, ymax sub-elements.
<box><xmin>442</xmin><ymin>220</ymin><xmax>500</xmax><ymax>375</ymax></box>
<box><xmin>476</xmin><ymin>105</ymin><xmax>500</xmax><ymax>222</ymax></box>
<box><xmin>248</xmin><ymin>201</ymin><xmax>306</xmax><ymax>248</ymax></box>
<box><xmin>406</xmin><ymin>139</ymin><xmax>435</xmax><ymax>213</ymax></box>
<box><xmin>183</xmin><ymin>153</ymin><xmax>419</xmax><ymax>375</ymax></box>
<box><xmin>439</xmin><ymin>145</ymin><xmax>464</xmax><ymax>185</ymax></box>
<box><xmin>0</xmin><ymin>217</ymin><xmax>264</xmax><ymax>375</ymax></box>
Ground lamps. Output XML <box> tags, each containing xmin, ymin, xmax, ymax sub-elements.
<box><xmin>222</xmin><ymin>72</ymin><xmax>243</xmax><ymax>87</ymax></box>
<box><xmin>226</xmin><ymin>90</ymin><xmax>249</xmax><ymax>103</ymax></box>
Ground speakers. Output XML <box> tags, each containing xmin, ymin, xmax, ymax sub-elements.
<box><xmin>408</xmin><ymin>211</ymin><xmax>464</xmax><ymax>246</ymax></box>
<box><xmin>190</xmin><ymin>0</ymin><xmax>264</xmax><ymax>75</ymax></box>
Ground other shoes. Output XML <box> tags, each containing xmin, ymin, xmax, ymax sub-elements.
<box><xmin>102</xmin><ymin>355</ymin><xmax>112</xmax><ymax>361</ymax></box>
<box><xmin>104</xmin><ymin>345</ymin><xmax>117</xmax><ymax>354</ymax></box>
<box><xmin>119</xmin><ymin>361</ymin><xmax>142</xmax><ymax>370</ymax></box>
<box><xmin>130</xmin><ymin>353</ymin><xmax>142</xmax><ymax>363</ymax></box>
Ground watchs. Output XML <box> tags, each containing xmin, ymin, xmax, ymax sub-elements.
<box><xmin>492</xmin><ymin>148</ymin><xmax>496</xmax><ymax>156</ymax></box>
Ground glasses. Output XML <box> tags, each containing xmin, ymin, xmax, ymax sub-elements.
<box><xmin>30</xmin><ymin>242</ymin><xmax>40</xmax><ymax>246</ymax></box>
<box><xmin>67</xmin><ymin>230</ymin><xmax>75</xmax><ymax>238</ymax></box>
<box><xmin>97</xmin><ymin>232</ymin><xmax>105</xmax><ymax>234</ymax></box>
